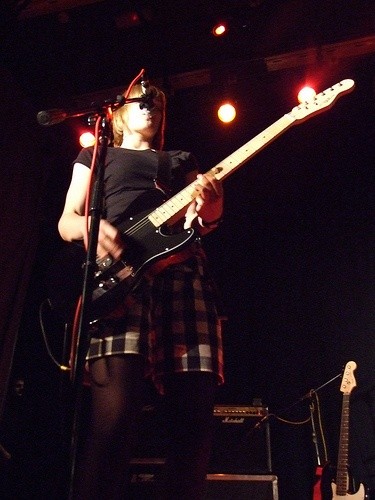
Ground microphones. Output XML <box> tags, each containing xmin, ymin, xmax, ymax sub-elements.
<box><xmin>139</xmin><ymin>87</ymin><xmax>157</xmax><ymax>109</ymax></box>
<box><xmin>239</xmin><ymin>422</ymin><xmax>262</xmax><ymax>448</ymax></box>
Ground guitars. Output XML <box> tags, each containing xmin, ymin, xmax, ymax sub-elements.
<box><xmin>328</xmin><ymin>359</ymin><xmax>368</xmax><ymax>499</ymax></box>
<box><xmin>37</xmin><ymin>77</ymin><xmax>355</xmax><ymax>373</ymax></box>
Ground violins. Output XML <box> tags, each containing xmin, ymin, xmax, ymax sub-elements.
<box><xmin>306</xmin><ymin>387</ymin><xmax>327</xmax><ymax>500</ymax></box>
